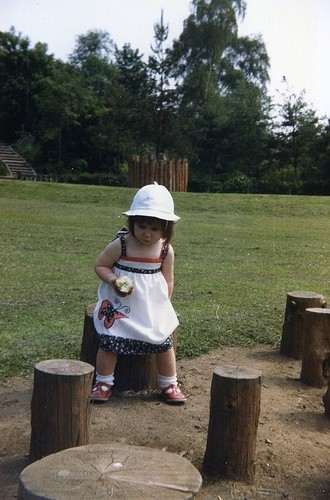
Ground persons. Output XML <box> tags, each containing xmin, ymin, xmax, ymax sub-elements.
<box><xmin>89</xmin><ymin>180</ymin><xmax>188</xmax><ymax>403</ymax></box>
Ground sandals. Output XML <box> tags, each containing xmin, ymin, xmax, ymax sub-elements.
<box><xmin>90</xmin><ymin>381</ymin><xmax>113</xmax><ymax>402</ymax></box>
<box><xmin>161</xmin><ymin>384</ymin><xmax>188</xmax><ymax>404</ymax></box>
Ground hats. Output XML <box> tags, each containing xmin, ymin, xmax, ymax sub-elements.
<box><xmin>121</xmin><ymin>181</ymin><xmax>181</xmax><ymax>224</ymax></box>
